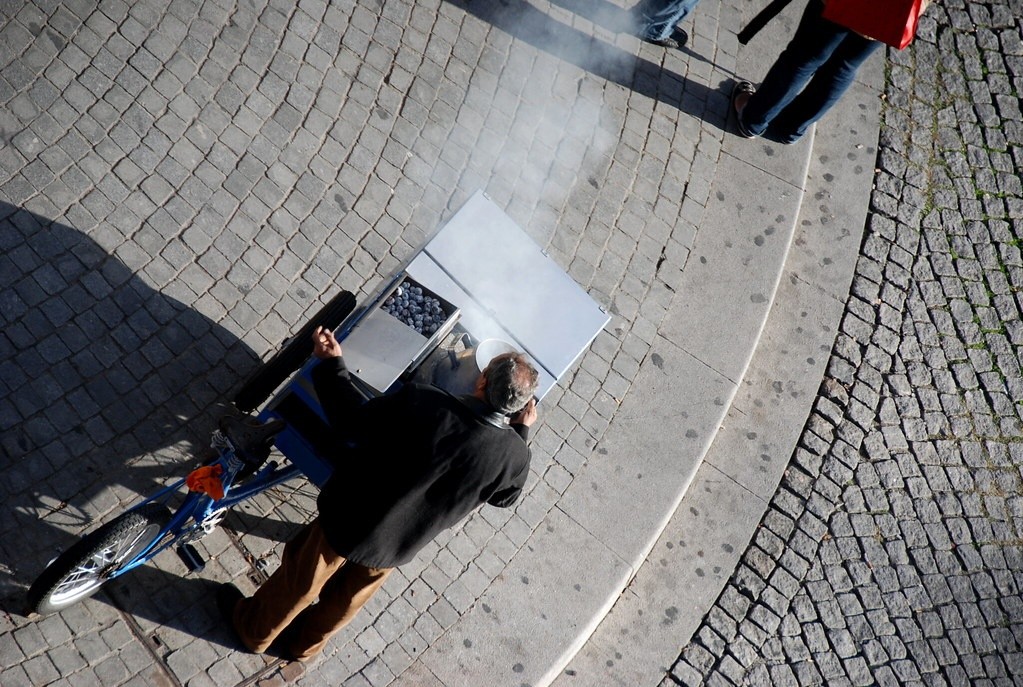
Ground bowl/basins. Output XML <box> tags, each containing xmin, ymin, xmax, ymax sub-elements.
<box><xmin>476</xmin><ymin>338</ymin><xmax>520</xmax><ymax>374</ymax></box>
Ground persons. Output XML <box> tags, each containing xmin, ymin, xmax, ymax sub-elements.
<box><xmin>218</xmin><ymin>324</ymin><xmax>540</xmax><ymax>662</ymax></box>
<box><xmin>621</xmin><ymin>0</ymin><xmax>700</xmax><ymax>47</ymax></box>
<box><xmin>732</xmin><ymin>0</ymin><xmax>933</xmax><ymax>145</ymax></box>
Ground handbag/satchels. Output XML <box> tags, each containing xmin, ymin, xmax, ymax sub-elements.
<box><xmin>823</xmin><ymin>0</ymin><xmax>921</xmax><ymax>49</ymax></box>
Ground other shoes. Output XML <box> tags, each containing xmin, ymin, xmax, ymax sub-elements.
<box><xmin>732</xmin><ymin>81</ymin><xmax>760</xmax><ymax>137</ymax></box>
<box><xmin>216</xmin><ymin>582</ymin><xmax>263</xmax><ymax>654</ymax></box>
<box><xmin>619</xmin><ymin>23</ymin><xmax>688</xmax><ymax>49</ymax></box>
<box><xmin>778</xmin><ymin>95</ymin><xmax>793</xmax><ymax>143</ymax></box>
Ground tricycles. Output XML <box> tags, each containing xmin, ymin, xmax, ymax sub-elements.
<box><xmin>24</xmin><ymin>189</ymin><xmax>611</xmax><ymax>617</ymax></box>
